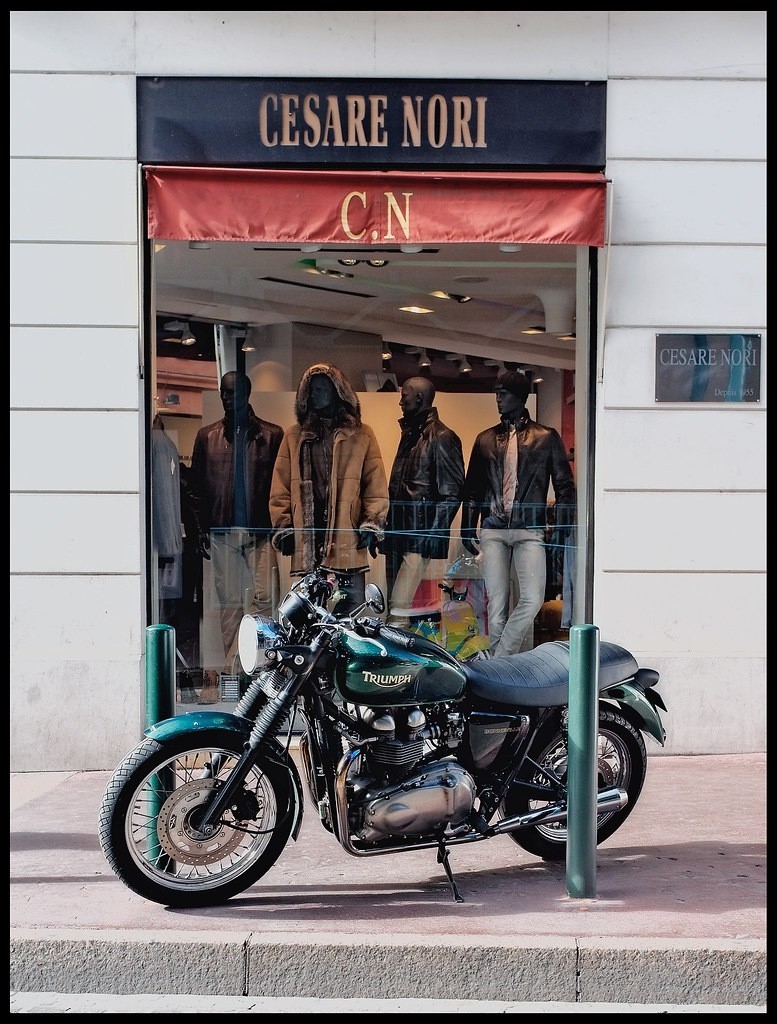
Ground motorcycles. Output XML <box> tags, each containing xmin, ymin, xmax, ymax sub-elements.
<box><xmin>97</xmin><ymin>559</ymin><xmax>669</xmax><ymax>910</ymax></box>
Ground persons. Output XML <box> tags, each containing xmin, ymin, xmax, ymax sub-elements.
<box><xmin>148</xmin><ymin>412</ymin><xmax>185</xmax><ymax>601</ymax></box>
<box><xmin>367</xmin><ymin>376</ymin><xmax>466</xmax><ymax>629</ymax></box>
<box><xmin>566</xmin><ymin>448</ymin><xmax>574</xmax><ymax>462</ymax></box>
<box><xmin>459</xmin><ymin>369</ymin><xmax>578</xmax><ymax>660</ymax></box>
<box><xmin>185</xmin><ymin>368</ymin><xmax>288</xmax><ymax>677</ymax></box>
<box><xmin>269</xmin><ymin>361</ymin><xmax>391</xmax><ymax>617</ymax></box>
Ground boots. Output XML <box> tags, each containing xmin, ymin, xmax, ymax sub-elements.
<box><xmin>198</xmin><ymin>669</ymin><xmax>220</xmax><ymax>705</ymax></box>
<box><xmin>179</xmin><ymin>668</ymin><xmax>198</xmax><ymax>704</ymax></box>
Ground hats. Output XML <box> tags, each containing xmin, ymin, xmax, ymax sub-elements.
<box><xmin>493</xmin><ymin>372</ymin><xmax>531</xmax><ymax>405</ymax></box>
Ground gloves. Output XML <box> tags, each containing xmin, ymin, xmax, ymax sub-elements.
<box><xmin>193</xmin><ymin>527</ymin><xmax>211</xmax><ymax>560</ymax></box>
<box><xmin>548</xmin><ymin>530</ymin><xmax>566</xmax><ymax>559</ymax></box>
<box><xmin>280</xmin><ymin>524</ymin><xmax>296</xmax><ymax>556</ymax></box>
<box><xmin>460</xmin><ymin>529</ymin><xmax>480</xmax><ymax>556</ymax></box>
<box><xmin>356</xmin><ymin>531</ymin><xmax>376</xmax><ymax>551</ymax></box>
<box><xmin>421</xmin><ymin>534</ymin><xmax>441</xmax><ymax>558</ymax></box>
<box><xmin>368</xmin><ymin>537</ymin><xmax>384</xmax><ymax>559</ymax></box>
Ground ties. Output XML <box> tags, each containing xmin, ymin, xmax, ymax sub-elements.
<box><xmin>503</xmin><ymin>424</ymin><xmax>518</xmax><ymax>520</ymax></box>
<box><xmin>233</xmin><ymin>432</ymin><xmax>248</xmax><ymax>527</ymax></box>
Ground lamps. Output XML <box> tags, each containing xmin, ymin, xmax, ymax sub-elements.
<box><xmin>154</xmin><ymin>308</ymin><xmax>547</xmax><ymax>395</ymax></box>
